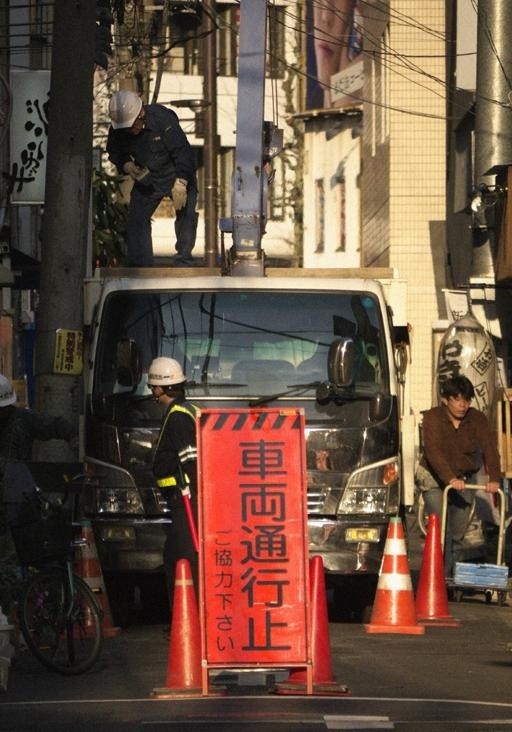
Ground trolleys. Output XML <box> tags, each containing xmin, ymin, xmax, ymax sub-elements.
<box><xmin>438</xmin><ymin>482</ymin><xmax>507</xmax><ymax>606</ymax></box>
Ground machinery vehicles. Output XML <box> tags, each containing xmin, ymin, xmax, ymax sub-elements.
<box><xmin>71</xmin><ymin>2</ymin><xmax>420</xmax><ymax>615</ymax></box>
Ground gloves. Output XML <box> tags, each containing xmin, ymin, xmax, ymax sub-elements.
<box><xmin>171</xmin><ymin>178</ymin><xmax>188</xmax><ymax>210</ymax></box>
<box><xmin>123</xmin><ymin>161</ymin><xmax>141</xmax><ymax>179</ymax></box>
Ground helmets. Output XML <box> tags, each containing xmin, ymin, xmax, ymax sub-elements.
<box><xmin>148</xmin><ymin>357</ymin><xmax>187</xmax><ymax>386</ymax></box>
<box><xmin>108</xmin><ymin>90</ymin><xmax>142</xmax><ymax>130</ymax></box>
<box><xmin>0</xmin><ymin>374</ymin><xmax>17</xmax><ymax>407</ymax></box>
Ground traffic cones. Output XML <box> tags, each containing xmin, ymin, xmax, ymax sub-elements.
<box><xmin>66</xmin><ymin>520</ymin><xmax>127</xmax><ymax>642</ymax></box>
<box><xmin>272</xmin><ymin>556</ymin><xmax>355</xmax><ymax>697</ymax></box>
<box><xmin>148</xmin><ymin>555</ymin><xmax>222</xmax><ymax>700</ymax></box>
<box><xmin>417</xmin><ymin>512</ymin><xmax>463</xmax><ymax>630</ymax></box>
<box><xmin>360</xmin><ymin>512</ymin><xmax>432</xmax><ymax>637</ymax></box>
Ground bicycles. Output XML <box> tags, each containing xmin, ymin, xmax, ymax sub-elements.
<box><xmin>16</xmin><ymin>468</ymin><xmax>109</xmax><ymax>673</ymax></box>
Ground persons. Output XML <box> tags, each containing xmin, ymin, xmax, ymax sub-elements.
<box><xmin>0</xmin><ymin>374</ymin><xmax>75</xmax><ymax>562</ymax></box>
<box><xmin>416</xmin><ymin>375</ymin><xmax>502</xmax><ymax>599</ymax></box>
<box><xmin>106</xmin><ymin>90</ymin><xmax>204</xmax><ymax>267</ymax></box>
<box><xmin>312</xmin><ymin>0</ymin><xmax>365</xmax><ymax>109</ymax></box>
<box><xmin>145</xmin><ymin>356</ymin><xmax>204</xmax><ymax>631</ymax></box>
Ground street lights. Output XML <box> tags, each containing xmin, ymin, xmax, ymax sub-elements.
<box><xmin>114</xmin><ymin>98</ymin><xmax>211</xmax><ymax>254</ymax></box>
